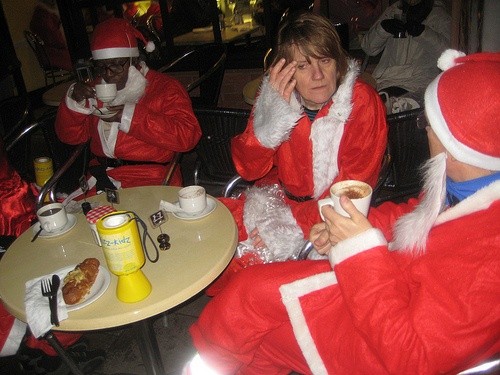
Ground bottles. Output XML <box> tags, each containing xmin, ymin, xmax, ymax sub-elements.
<box><xmin>87</xmin><ymin>205</ymin><xmax>117</xmax><ymax>248</ymax></box>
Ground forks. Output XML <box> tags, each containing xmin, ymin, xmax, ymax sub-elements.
<box><xmin>41</xmin><ymin>279</ymin><xmax>59</xmax><ymax>326</ymax></box>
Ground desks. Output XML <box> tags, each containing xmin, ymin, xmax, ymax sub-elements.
<box><xmin>41</xmin><ymin>81</ymin><xmax>72</xmax><ymax>105</ymax></box>
<box><xmin>0</xmin><ymin>185</ymin><xmax>239</xmax><ymax>374</ymax></box>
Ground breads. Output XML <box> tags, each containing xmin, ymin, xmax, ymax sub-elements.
<box><xmin>62</xmin><ymin>257</ymin><xmax>100</xmax><ymax>305</ymax></box>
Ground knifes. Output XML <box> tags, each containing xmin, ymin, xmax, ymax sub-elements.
<box><xmin>51</xmin><ymin>275</ymin><xmax>60</xmax><ymax>325</ymax></box>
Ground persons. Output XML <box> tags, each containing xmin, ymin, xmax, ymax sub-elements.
<box><xmin>203</xmin><ymin>14</ymin><xmax>388</xmax><ymax>295</ymax></box>
<box><xmin>30</xmin><ymin>0</ymin><xmax>72</xmax><ymax>69</ymax></box>
<box><xmin>0</xmin><ymin>159</ymin><xmax>105</xmax><ymax>375</ymax></box>
<box><xmin>55</xmin><ymin>18</ymin><xmax>201</xmax><ymax>205</ymax></box>
<box><xmin>361</xmin><ymin>0</ymin><xmax>452</xmax><ymax>112</ymax></box>
<box><xmin>184</xmin><ymin>50</ymin><xmax>500</xmax><ymax>375</ymax></box>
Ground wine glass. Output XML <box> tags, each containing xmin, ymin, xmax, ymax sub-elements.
<box><xmin>393</xmin><ymin>6</ymin><xmax>407</xmax><ymax>38</ymax></box>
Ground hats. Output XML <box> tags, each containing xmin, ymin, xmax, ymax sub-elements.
<box><xmin>424</xmin><ymin>49</ymin><xmax>500</xmax><ymax>173</ymax></box>
<box><xmin>90</xmin><ymin>17</ymin><xmax>156</xmax><ymax>61</ymax></box>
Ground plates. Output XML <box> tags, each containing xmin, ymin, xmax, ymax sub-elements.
<box><xmin>92</xmin><ymin>108</ymin><xmax>120</xmax><ymax>119</ymax></box>
<box><xmin>170</xmin><ymin>196</ymin><xmax>218</xmax><ymax>221</ymax></box>
<box><xmin>32</xmin><ymin>213</ymin><xmax>78</xmax><ymax>238</ymax></box>
<box><xmin>51</xmin><ymin>264</ymin><xmax>111</xmax><ymax>313</ymax></box>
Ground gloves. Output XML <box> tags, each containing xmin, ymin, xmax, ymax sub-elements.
<box><xmin>406</xmin><ymin>18</ymin><xmax>425</xmax><ymax>36</ymax></box>
<box><xmin>381</xmin><ymin>19</ymin><xmax>404</xmax><ymax>34</ymax></box>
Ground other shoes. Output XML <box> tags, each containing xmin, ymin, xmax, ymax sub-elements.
<box><xmin>17</xmin><ymin>340</ymin><xmax>107</xmax><ymax>375</ymax></box>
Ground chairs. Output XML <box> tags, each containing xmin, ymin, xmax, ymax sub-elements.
<box><xmin>0</xmin><ymin>31</ymin><xmax>430</xmax><ymax>259</ymax></box>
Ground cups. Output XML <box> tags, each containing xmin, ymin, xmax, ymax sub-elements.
<box><xmin>90</xmin><ymin>83</ymin><xmax>118</xmax><ymax>102</ymax></box>
<box><xmin>37</xmin><ymin>202</ymin><xmax>67</xmax><ymax>233</ymax></box>
<box><xmin>177</xmin><ymin>185</ymin><xmax>207</xmax><ymax>212</ymax></box>
<box><xmin>317</xmin><ymin>179</ymin><xmax>373</xmax><ymax>221</ymax></box>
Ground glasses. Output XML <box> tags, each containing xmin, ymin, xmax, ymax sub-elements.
<box><xmin>91</xmin><ymin>59</ymin><xmax>129</xmax><ymax>76</ymax></box>
